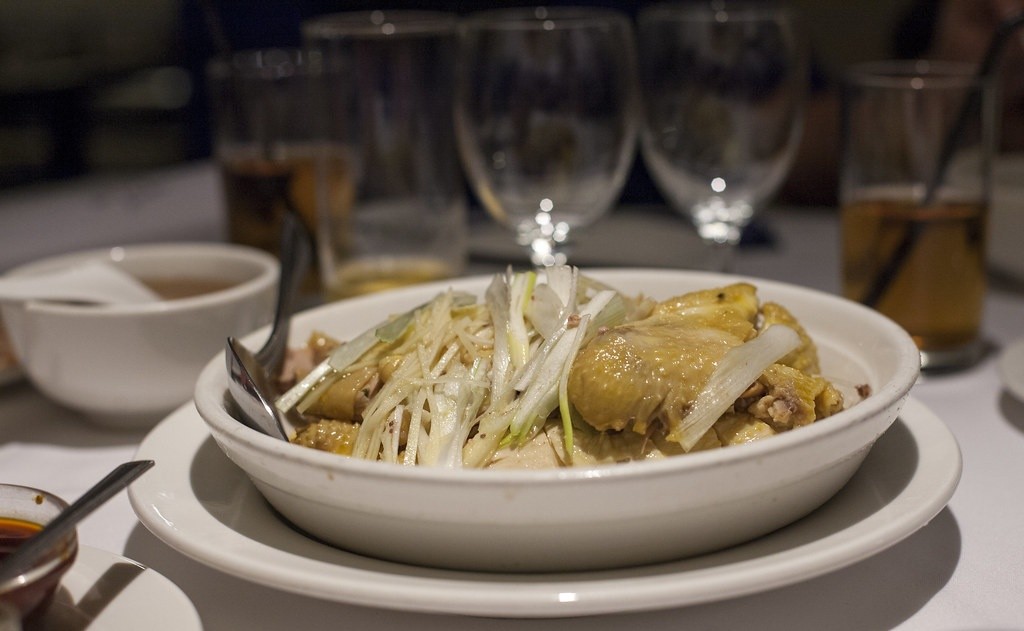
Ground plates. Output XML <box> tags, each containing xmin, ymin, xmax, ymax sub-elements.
<box><xmin>0</xmin><ymin>543</ymin><xmax>204</xmax><ymax>631</ymax></box>
<box><xmin>127</xmin><ymin>394</ymin><xmax>963</xmax><ymax>615</ymax></box>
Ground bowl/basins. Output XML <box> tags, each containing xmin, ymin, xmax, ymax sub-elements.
<box><xmin>193</xmin><ymin>268</ymin><xmax>921</xmax><ymax>570</ymax></box>
<box><xmin>0</xmin><ymin>484</ymin><xmax>77</xmax><ymax>623</ymax></box>
<box><xmin>0</xmin><ymin>240</ymin><xmax>282</xmax><ymax>426</ymax></box>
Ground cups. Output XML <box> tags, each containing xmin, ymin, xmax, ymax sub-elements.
<box><xmin>302</xmin><ymin>11</ymin><xmax>458</xmax><ymax>295</ymax></box>
<box><xmin>209</xmin><ymin>48</ymin><xmax>333</xmax><ymax>313</ymax></box>
<box><xmin>840</xmin><ymin>59</ymin><xmax>1003</xmax><ymax>369</ymax></box>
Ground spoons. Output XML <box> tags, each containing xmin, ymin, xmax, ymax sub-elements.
<box><xmin>226</xmin><ymin>228</ymin><xmax>307</xmax><ymax>441</ymax></box>
<box><xmin>0</xmin><ymin>260</ymin><xmax>165</xmax><ymax>304</ymax></box>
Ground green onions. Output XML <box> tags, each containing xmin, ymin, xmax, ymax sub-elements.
<box><xmin>274</xmin><ymin>257</ymin><xmax>802</xmax><ymax>470</ymax></box>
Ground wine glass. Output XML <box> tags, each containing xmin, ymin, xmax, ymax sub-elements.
<box><xmin>638</xmin><ymin>8</ymin><xmax>808</xmax><ymax>273</ymax></box>
<box><xmin>443</xmin><ymin>9</ymin><xmax>638</xmax><ymax>266</ymax></box>
